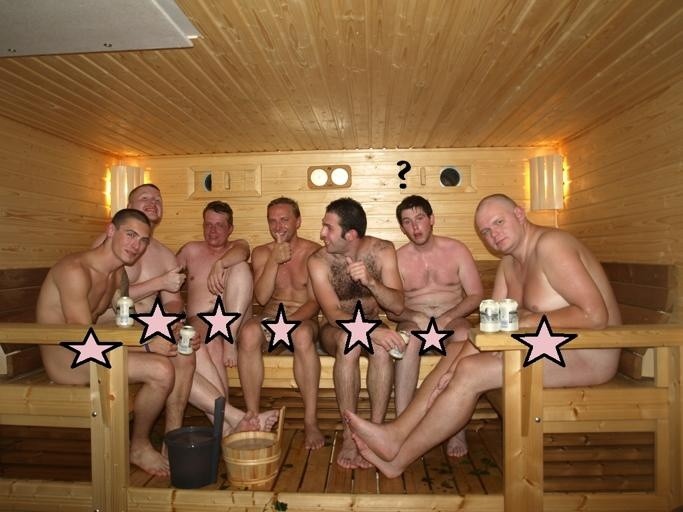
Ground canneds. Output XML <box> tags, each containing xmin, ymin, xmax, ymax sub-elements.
<box><xmin>499</xmin><ymin>298</ymin><xmax>519</xmax><ymax>332</ymax></box>
<box><xmin>115</xmin><ymin>296</ymin><xmax>135</xmax><ymax>328</ymax></box>
<box><xmin>479</xmin><ymin>299</ymin><xmax>501</xmax><ymax>332</ymax></box>
<box><xmin>260</xmin><ymin>317</ymin><xmax>275</xmax><ymax>342</ymax></box>
<box><xmin>177</xmin><ymin>325</ymin><xmax>196</xmax><ymax>355</ymax></box>
<box><xmin>388</xmin><ymin>330</ymin><xmax>410</xmax><ymax>359</ymax></box>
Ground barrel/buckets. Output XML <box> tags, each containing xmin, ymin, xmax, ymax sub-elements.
<box><xmin>218</xmin><ymin>406</ymin><xmax>287</xmax><ymax>492</ymax></box>
<box><xmin>163</xmin><ymin>396</ymin><xmax>224</xmax><ymax>490</ymax></box>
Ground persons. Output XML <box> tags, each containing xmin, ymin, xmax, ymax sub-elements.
<box><xmin>87</xmin><ymin>180</ymin><xmax>280</xmax><ymax>444</ymax></box>
<box><xmin>304</xmin><ymin>195</ymin><xmax>408</xmax><ymax>470</ymax></box>
<box><xmin>232</xmin><ymin>195</ymin><xmax>326</xmax><ymax>450</ymax></box>
<box><xmin>34</xmin><ymin>206</ymin><xmax>201</xmax><ymax>482</ymax></box>
<box><xmin>384</xmin><ymin>194</ymin><xmax>486</xmax><ymax>459</ymax></box>
<box><xmin>340</xmin><ymin>192</ymin><xmax>623</xmax><ymax>481</ymax></box>
<box><xmin>174</xmin><ymin>198</ymin><xmax>256</xmax><ymax>406</ymax></box>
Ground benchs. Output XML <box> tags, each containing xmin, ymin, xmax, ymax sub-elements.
<box><xmin>2</xmin><ymin>261</ymin><xmax>675</xmax><ymax>429</ymax></box>
